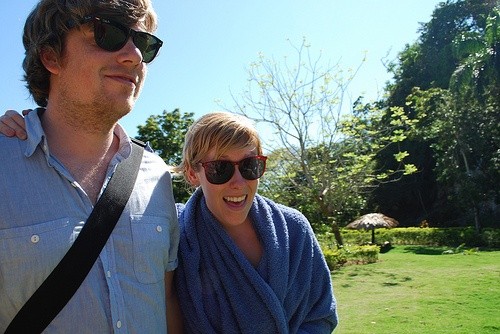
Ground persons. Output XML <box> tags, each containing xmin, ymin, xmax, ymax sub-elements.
<box><xmin>0</xmin><ymin>108</ymin><xmax>338</xmax><ymax>334</ymax></box>
<box><xmin>0</xmin><ymin>0</ymin><xmax>179</xmax><ymax>334</ymax></box>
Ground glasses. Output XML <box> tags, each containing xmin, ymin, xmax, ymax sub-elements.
<box><xmin>66</xmin><ymin>16</ymin><xmax>163</xmax><ymax>63</ymax></box>
<box><xmin>195</xmin><ymin>156</ymin><xmax>268</xmax><ymax>185</ymax></box>
